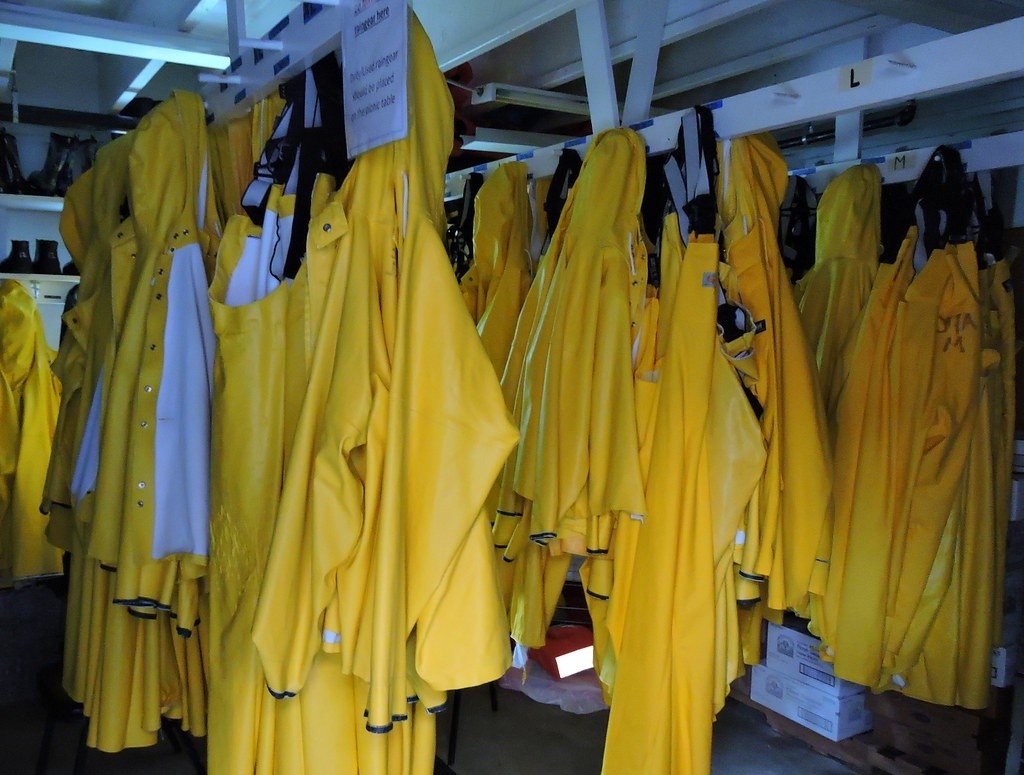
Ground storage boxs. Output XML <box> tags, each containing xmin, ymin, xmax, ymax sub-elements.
<box><xmin>864</xmin><ymin>686</ymin><xmax>1013</xmax><ymax>752</ymax></box>
<box><xmin>750</xmin><ymin>658</ymin><xmax>874</xmax><ymax>741</ymax></box>
<box><xmin>955</xmin><ymin>436</ymin><xmax>1024</xmax><ymax>719</ymax></box>
<box><xmin>873</xmin><ymin>715</ymin><xmax>1011</xmax><ymax>775</ymax></box>
<box><xmin>767</xmin><ymin>622</ymin><xmax>866</xmax><ymax>698</ymax></box>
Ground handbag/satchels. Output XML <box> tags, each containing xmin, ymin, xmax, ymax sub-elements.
<box><xmin>528</xmin><ymin>623</ymin><xmax>595</xmax><ymax>680</ymax></box>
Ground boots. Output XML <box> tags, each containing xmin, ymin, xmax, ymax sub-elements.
<box><xmin>27</xmin><ymin>132</ymin><xmax>73</xmax><ymax>196</ymax></box>
<box><xmin>55</xmin><ymin>135</ymin><xmax>97</xmax><ymax>197</ymax></box>
<box><xmin>0</xmin><ymin>240</ymin><xmax>31</xmax><ymax>273</ymax></box>
<box><xmin>0</xmin><ymin>127</ymin><xmax>28</xmax><ymax>194</ymax></box>
<box><xmin>32</xmin><ymin>239</ymin><xmax>61</xmax><ymax>274</ymax></box>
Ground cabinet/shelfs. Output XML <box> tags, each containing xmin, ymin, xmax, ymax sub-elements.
<box><xmin>0</xmin><ymin>193</ymin><xmax>81</xmax><ymax>303</ymax></box>
